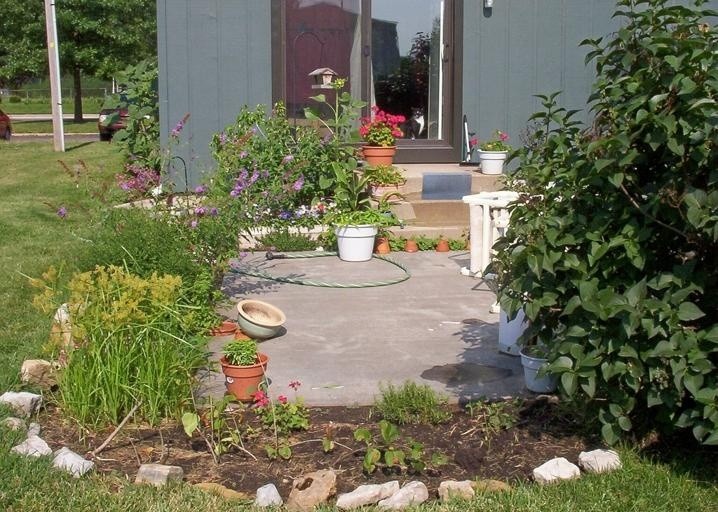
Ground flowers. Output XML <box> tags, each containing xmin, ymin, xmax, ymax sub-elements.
<box><xmin>359</xmin><ymin>105</ymin><xmax>406</xmax><ymax>146</ymax></box>
<box><xmin>471</xmin><ymin>130</ymin><xmax>513</xmax><ymax>152</ymax></box>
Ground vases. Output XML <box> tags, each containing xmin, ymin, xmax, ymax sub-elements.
<box><xmin>236</xmin><ymin>299</ymin><xmax>287</xmax><ymax>339</ymax></box>
<box><xmin>478</xmin><ymin>149</ymin><xmax>508</xmax><ymax>175</ymax></box>
<box><xmin>363</xmin><ymin>145</ymin><xmax>397</xmax><ymax>170</ymax></box>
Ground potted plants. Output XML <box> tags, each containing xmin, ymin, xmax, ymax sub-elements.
<box><xmin>520</xmin><ymin>345</ymin><xmax>562</xmax><ymax>393</ymax></box>
<box><xmin>220</xmin><ymin>340</ymin><xmax>270</xmax><ymax>407</ymax></box>
<box><xmin>331</xmin><ymin>207</ymin><xmax>381</xmax><ymax>262</ymax></box>
<box><xmin>370</xmin><ymin>166</ymin><xmax>407</xmax><ymax>205</ymax></box>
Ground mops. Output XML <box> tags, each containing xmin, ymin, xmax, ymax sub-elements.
<box><xmin>460</xmin><ymin>114</ymin><xmax>480</xmax><ymax>167</ymax></box>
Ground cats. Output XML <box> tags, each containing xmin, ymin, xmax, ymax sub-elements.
<box><xmin>399</xmin><ymin>106</ymin><xmax>426</xmax><ymax>141</ymax></box>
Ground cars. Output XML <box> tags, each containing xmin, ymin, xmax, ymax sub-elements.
<box><xmin>0</xmin><ymin>109</ymin><xmax>13</xmax><ymax>141</ymax></box>
<box><xmin>98</xmin><ymin>92</ymin><xmax>152</xmax><ymax>140</ymax></box>
